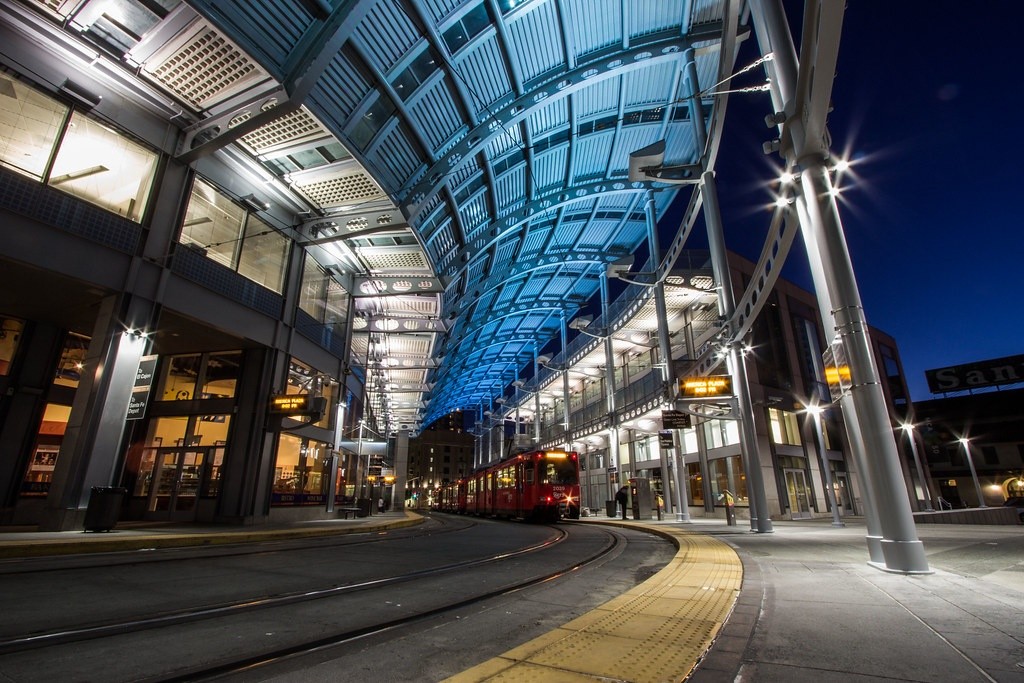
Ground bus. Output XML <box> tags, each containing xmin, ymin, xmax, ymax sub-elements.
<box><xmin>431</xmin><ymin>449</ymin><xmax>581</xmax><ymax>522</ymax></box>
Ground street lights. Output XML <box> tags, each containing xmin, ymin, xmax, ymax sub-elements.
<box><xmin>958</xmin><ymin>438</ymin><xmax>989</xmax><ymax>508</ymax></box>
<box><xmin>902</xmin><ymin>424</ymin><xmax>936</xmax><ymax>513</ymax></box>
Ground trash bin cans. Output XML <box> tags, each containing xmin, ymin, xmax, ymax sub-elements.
<box><xmin>606</xmin><ymin>500</ymin><xmax>617</xmax><ymax>517</ymax></box>
<box><xmin>357</xmin><ymin>498</ymin><xmax>370</xmax><ymax>517</ymax></box>
<box><xmin>83</xmin><ymin>486</ymin><xmax>126</xmax><ymax>532</ymax></box>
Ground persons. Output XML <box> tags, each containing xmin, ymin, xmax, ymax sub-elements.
<box><xmin>615</xmin><ymin>484</ymin><xmax>629</xmax><ymax>520</ymax></box>
<box><xmin>378</xmin><ymin>497</ymin><xmax>385</xmax><ymax>513</ymax></box>
